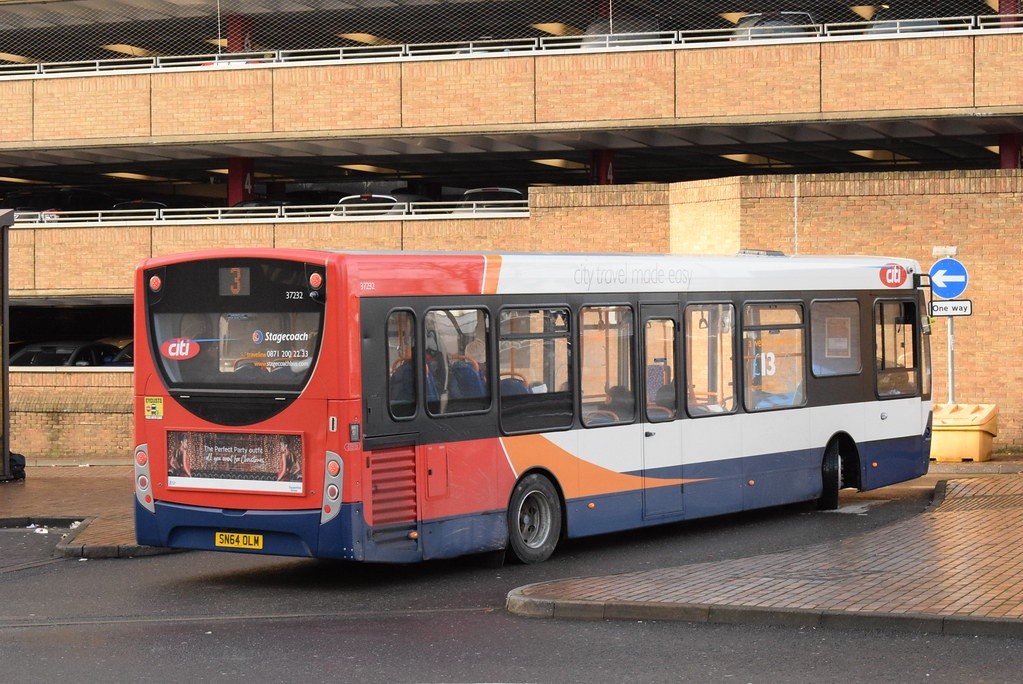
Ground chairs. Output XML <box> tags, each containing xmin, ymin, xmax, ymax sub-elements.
<box><xmin>389</xmin><ymin>354</ymin><xmax>711</xmax><ymax>427</ymax></box>
<box><xmin>234</xmin><ymin>360</ymin><xmax>308</xmax><ymax>385</ymax></box>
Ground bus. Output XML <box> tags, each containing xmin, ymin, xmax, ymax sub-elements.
<box><xmin>130</xmin><ymin>245</ymin><xmax>935</xmax><ymax>564</ymax></box>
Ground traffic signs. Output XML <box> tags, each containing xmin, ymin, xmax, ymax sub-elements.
<box><xmin>928</xmin><ymin>258</ymin><xmax>973</xmax><ymax>317</ymax></box>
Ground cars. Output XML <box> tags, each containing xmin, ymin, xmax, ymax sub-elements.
<box><xmin>96</xmin><ymin>337</ymin><xmax>133</xmax><ymax>364</ymax></box>
<box><xmin>726</xmin><ymin>12</ymin><xmax>820</xmax><ymax>38</ymax></box>
<box><xmin>455</xmin><ymin>187</ymin><xmax>526</xmax><ymax>213</ymax></box>
<box><xmin>865</xmin><ymin>9</ymin><xmax>940</xmax><ymax>33</ymax></box>
<box><xmin>9</xmin><ymin>341</ymin><xmax>100</xmax><ymax>367</ymax></box>
<box><xmin>219</xmin><ymin>200</ymin><xmax>297</xmax><ymax>219</ymax></box>
<box><xmin>331</xmin><ymin>193</ymin><xmax>450</xmax><ymax>217</ymax></box>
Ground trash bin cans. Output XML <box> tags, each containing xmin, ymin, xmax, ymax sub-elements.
<box><xmin>928</xmin><ymin>404</ymin><xmax>999</xmax><ymax>463</ymax></box>
